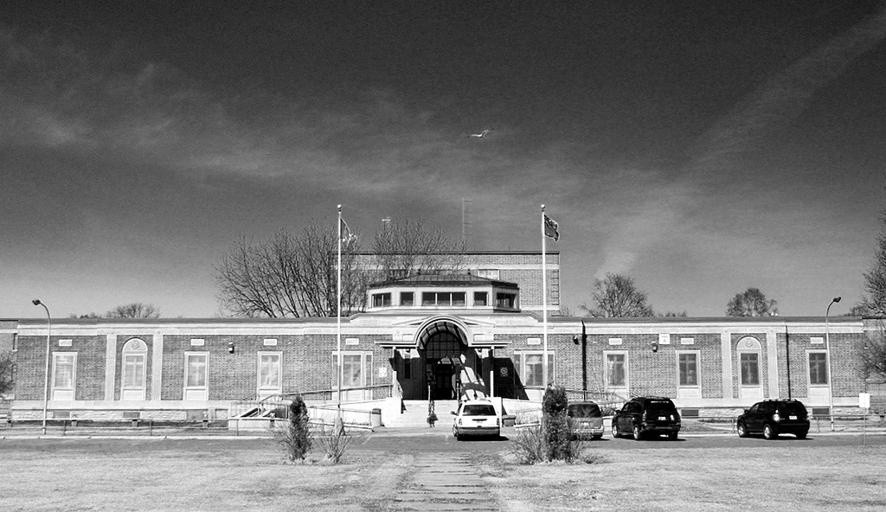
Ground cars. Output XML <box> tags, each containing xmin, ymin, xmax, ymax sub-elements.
<box><xmin>737</xmin><ymin>399</ymin><xmax>810</xmax><ymax>440</ymax></box>
<box><xmin>450</xmin><ymin>400</ymin><xmax>500</xmax><ymax>440</ymax></box>
<box><xmin>567</xmin><ymin>396</ymin><xmax>681</xmax><ymax>441</ymax></box>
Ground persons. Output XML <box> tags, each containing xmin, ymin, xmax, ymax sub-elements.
<box><xmin>425</xmin><ymin>400</ymin><xmax>438</xmax><ymax>427</ymax></box>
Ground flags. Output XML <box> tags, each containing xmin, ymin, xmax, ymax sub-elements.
<box><xmin>341</xmin><ymin>218</ymin><xmax>357</xmax><ymax>254</ymax></box>
<box><xmin>544</xmin><ymin>214</ymin><xmax>559</xmax><ymax>242</ymax></box>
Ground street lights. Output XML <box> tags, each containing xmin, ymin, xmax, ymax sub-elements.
<box><xmin>32</xmin><ymin>299</ymin><xmax>51</xmax><ymax>435</ymax></box>
<box><xmin>825</xmin><ymin>297</ymin><xmax>842</xmax><ymax>432</ymax></box>
